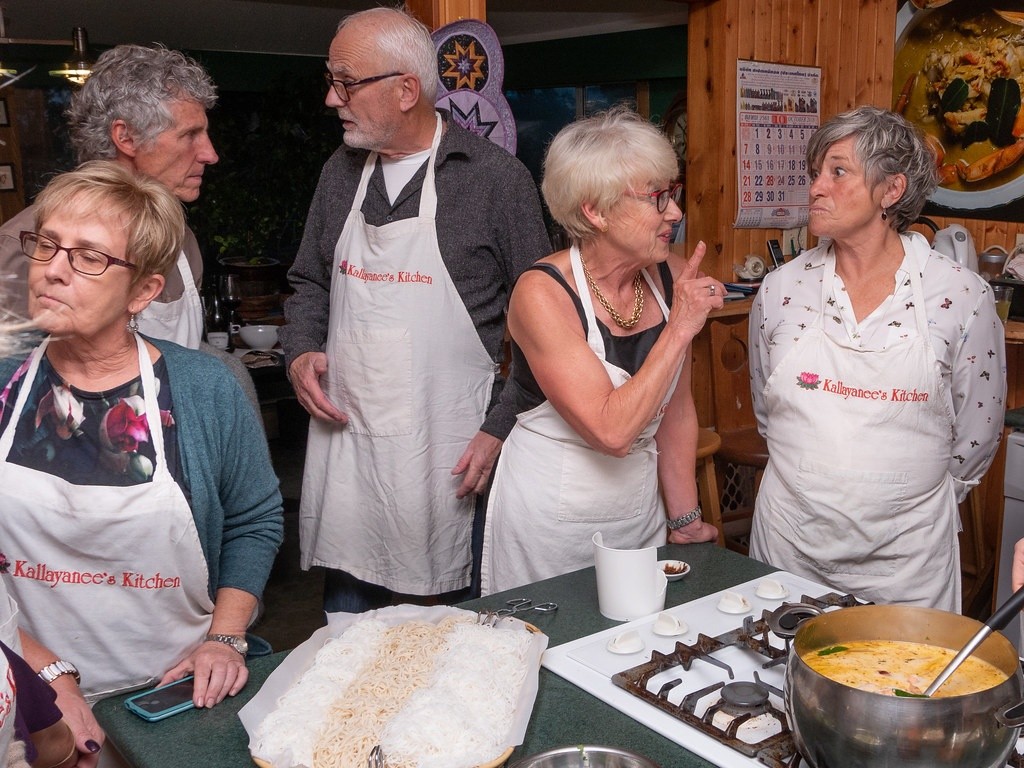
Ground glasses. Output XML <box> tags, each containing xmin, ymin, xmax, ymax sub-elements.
<box><xmin>325</xmin><ymin>72</ymin><xmax>406</xmax><ymax>102</ymax></box>
<box><xmin>622</xmin><ymin>183</ymin><xmax>683</xmax><ymax>214</ymax></box>
<box><xmin>19</xmin><ymin>230</ymin><xmax>155</xmax><ymax>276</ymax></box>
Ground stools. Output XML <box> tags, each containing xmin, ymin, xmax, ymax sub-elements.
<box><xmin>658</xmin><ymin>428</ymin><xmax>725</xmax><ymax>551</ymax></box>
<box><xmin>717</xmin><ymin>426</ymin><xmax>770</xmax><ymax>502</ymax></box>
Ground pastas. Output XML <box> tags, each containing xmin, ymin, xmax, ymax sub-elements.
<box><xmin>310</xmin><ymin>616</ymin><xmax>465</xmax><ymax>768</ymax></box>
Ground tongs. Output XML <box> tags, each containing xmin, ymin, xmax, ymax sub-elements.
<box><xmin>477</xmin><ymin>598</ymin><xmax>558</xmax><ymax>628</ymax></box>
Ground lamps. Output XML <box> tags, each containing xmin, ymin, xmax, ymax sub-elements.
<box><xmin>49</xmin><ymin>27</ymin><xmax>94</xmax><ymax>86</ymax></box>
<box><xmin>0</xmin><ymin>60</ymin><xmax>36</xmax><ymax>89</ymax></box>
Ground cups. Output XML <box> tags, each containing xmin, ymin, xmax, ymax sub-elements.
<box><xmin>980</xmin><ymin>252</ymin><xmax>1007</xmax><ymax>281</ymax></box>
<box><xmin>990</xmin><ymin>285</ymin><xmax>1014</xmax><ymax>323</ymax></box>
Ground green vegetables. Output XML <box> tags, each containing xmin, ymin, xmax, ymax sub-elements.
<box><xmin>817</xmin><ymin>646</ymin><xmax>931</xmax><ymax>699</ymax></box>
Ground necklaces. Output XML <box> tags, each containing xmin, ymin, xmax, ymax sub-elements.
<box><xmin>578</xmin><ymin>249</ymin><xmax>645</xmax><ymax>329</ymax></box>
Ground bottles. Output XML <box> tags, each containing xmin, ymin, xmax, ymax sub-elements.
<box><xmin>206</xmin><ymin>272</ymin><xmax>235</xmax><ymax>353</ymax></box>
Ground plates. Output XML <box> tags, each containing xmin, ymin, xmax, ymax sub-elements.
<box><xmin>658</xmin><ymin>560</ymin><xmax>691</xmax><ymax>581</ymax></box>
<box><xmin>896</xmin><ymin>0</ymin><xmax>1024</xmax><ymax>211</ymax></box>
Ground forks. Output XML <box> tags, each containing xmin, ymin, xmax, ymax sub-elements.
<box><xmin>368</xmin><ymin>745</ymin><xmax>383</xmax><ymax>768</ymax></box>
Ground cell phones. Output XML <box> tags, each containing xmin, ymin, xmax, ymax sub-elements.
<box><xmin>124</xmin><ymin>675</ymin><xmax>196</xmax><ymax>722</ymax></box>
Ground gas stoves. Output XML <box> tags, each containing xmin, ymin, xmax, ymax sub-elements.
<box><xmin>540</xmin><ymin>571</ymin><xmax>1024</xmax><ymax>768</ymax></box>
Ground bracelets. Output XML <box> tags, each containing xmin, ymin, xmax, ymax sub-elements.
<box><xmin>667</xmin><ymin>505</ymin><xmax>702</xmax><ymax>529</ymax></box>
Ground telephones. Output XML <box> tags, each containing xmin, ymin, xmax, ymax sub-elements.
<box><xmin>766</xmin><ymin>239</ymin><xmax>785</xmax><ymax>272</ymax></box>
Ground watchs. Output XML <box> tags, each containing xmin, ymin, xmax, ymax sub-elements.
<box><xmin>37</xmin><ymin>660</ymin><xmax>81</xmax><ymax>688</ymax></box>
<box><xmin>203</xmin><ymin>634</ymin><xmax>247</xmax><ymax>659</ymax></box>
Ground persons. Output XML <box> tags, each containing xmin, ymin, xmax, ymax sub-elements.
<box><xmin>747</xmin><ymin>104</ymin><xmax>1009</xmax><ymax>617</ymax></box>
<box><xmin>0</xmin><ymin>159</ymin><xmax>286</xmax><ymax>768</ymax></box>
<box><xmin>0</xmin><ymin>43</ymin><xmax>219</xmax><ymax>351</ymax></box>
<box><xmin>468</xmin><ymin>110</ymin><xmax>721</xmax><ymax>596</ymax></box>
<box><xmin>277</xmin><ymin>6</ymin><xmax>557</xmax><ymax>618</ymax></box>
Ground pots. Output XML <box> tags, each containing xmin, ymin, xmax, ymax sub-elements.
<box><xmin>768</xmin><ymin>603</ymin><xmax>1024</xmax><ymax>768</ymax></box>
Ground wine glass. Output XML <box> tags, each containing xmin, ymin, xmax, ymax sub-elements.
<box><xmin>219</xmin><ymin>274</ymin><xmax>241</xmax><ymax>325</ymax></box>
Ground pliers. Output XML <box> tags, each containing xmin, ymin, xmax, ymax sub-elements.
<box><xmin>481</xmin><ymin>597</ymin><xmax>557</xmax><ymax>626</ymax></box>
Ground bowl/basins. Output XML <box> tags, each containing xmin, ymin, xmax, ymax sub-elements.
<box><xmin>231</xmin><ymin>324</ymin><xmax>283</xmax><ymax>351</ymax></box>
<box><xmin>508</xmin><ymin>743</ymin><xmax>662</xmax><ymax>768</ymax></box>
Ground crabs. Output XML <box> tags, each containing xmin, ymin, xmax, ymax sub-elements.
<box><xmin>892</xmin><ymin>8</ymin><xmax>1023</xmax><ymax>191</ymax></box>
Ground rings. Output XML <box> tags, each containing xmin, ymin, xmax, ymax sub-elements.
<box><xmin>709</xmin><ymin>284</ymin><xmax>715</xmax><ymax>297</ymax></box>
